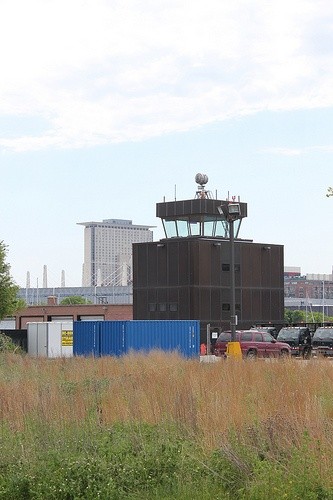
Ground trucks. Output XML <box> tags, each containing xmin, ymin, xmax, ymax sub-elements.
<box><xmin>311</xmin><ymin>326</ymin><xmax>333</xmax><ymax>358</ymax></box>
<box><xmin>277</xmin><ymin>326</ymin><xmax>312</xmax><ymax>358</ymax></box>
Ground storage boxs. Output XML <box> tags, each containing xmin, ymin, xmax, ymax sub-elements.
<box><xmin>24</xmin><ymin>319</ymin><xmax>202</xmax><ymax>364</ymax></box>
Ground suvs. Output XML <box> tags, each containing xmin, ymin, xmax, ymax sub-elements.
<box><xmin>212</xmin><ymin>330</ymin><xmax>292</xmax><ymax>361</ymax></box>
